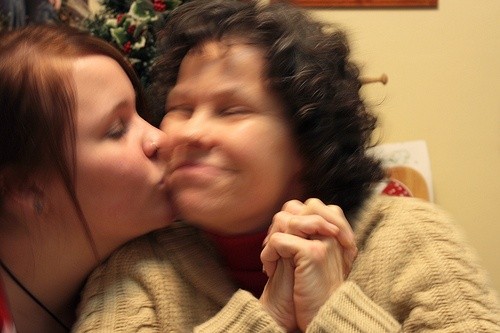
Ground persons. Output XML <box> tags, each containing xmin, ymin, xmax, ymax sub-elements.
<box><xmin>72</xmin><ymin>0</ymin><xmax>500</xmax><ymax>333</ymax></box>
<box><xmin>0</xmin><ymin>24</ymin><xmax>174</xmax><ymax>333</ymax></box>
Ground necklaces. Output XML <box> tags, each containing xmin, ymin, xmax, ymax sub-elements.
<box><xmin>0</xmin><ymin>259</ymin><xmax>72</xmax><ymax>333</ymax></box>
